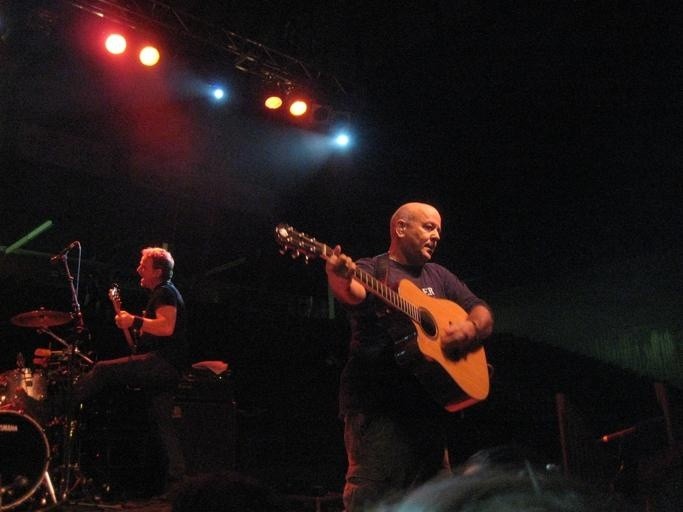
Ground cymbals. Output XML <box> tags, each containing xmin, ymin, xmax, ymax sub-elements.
<box><xmin>11</xmin><ymin>310</ymin><xmax>72</xmax><ymax>328</ymax></box>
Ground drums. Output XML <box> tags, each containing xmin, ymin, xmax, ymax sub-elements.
<box><xmin>47</xmin><ymin>362</ymin><xmax>79</xmax><ymax>381</ymax></box>
<box><xmin>0</xmin><ymin>368</ymin><xmax>51</xmax><ymax>402</ymax></box>
<box><xmin>0</xmin><ymin>390</ymin><xmax>52</xmax><ymax>509</ymax></box>
<box><xmin>45</xmin><ymin>414</ymin><xmax>107</xmax><ymax>477</ymax></box>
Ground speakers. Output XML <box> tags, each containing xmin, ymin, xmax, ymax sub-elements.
<box><xmin>167</xmin><ymin>402</ymin><xmax>240</xmax><ymax>476</ymax></box>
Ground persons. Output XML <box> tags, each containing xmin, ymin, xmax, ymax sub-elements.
<box><xmin>336</xmin><ymin>200</ymin><xmax>494</xmax><ymax>512</ymax></box>
<box><xmin>44</xmin><ymin>243</ymin><xmax>186</xmax><ymax>500</ymax></box>
<box><xmin>163</xmin><ymin>437</ymin><xmax>610</xmax><ymax>512</ymax></box>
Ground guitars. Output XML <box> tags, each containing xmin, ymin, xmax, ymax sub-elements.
<box><xmin>109</xmin><ymin>287</ymin><xmax>147</xmax><ymax>386</ymax></box>
<box><xmin>275</xmin><ymin>224</ymin><xmax>490</xmax><ymax>412</ymax></box>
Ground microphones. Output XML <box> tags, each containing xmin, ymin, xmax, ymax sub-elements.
<box><xmin>50</xmin><ymin>240</ymin><xmax>76</xmax><ymax>264</ymax></box>
<box><xmin>601</xmin><ymin>426</ymin><xmax>639</xmax><ymax>444</ymax></box>
